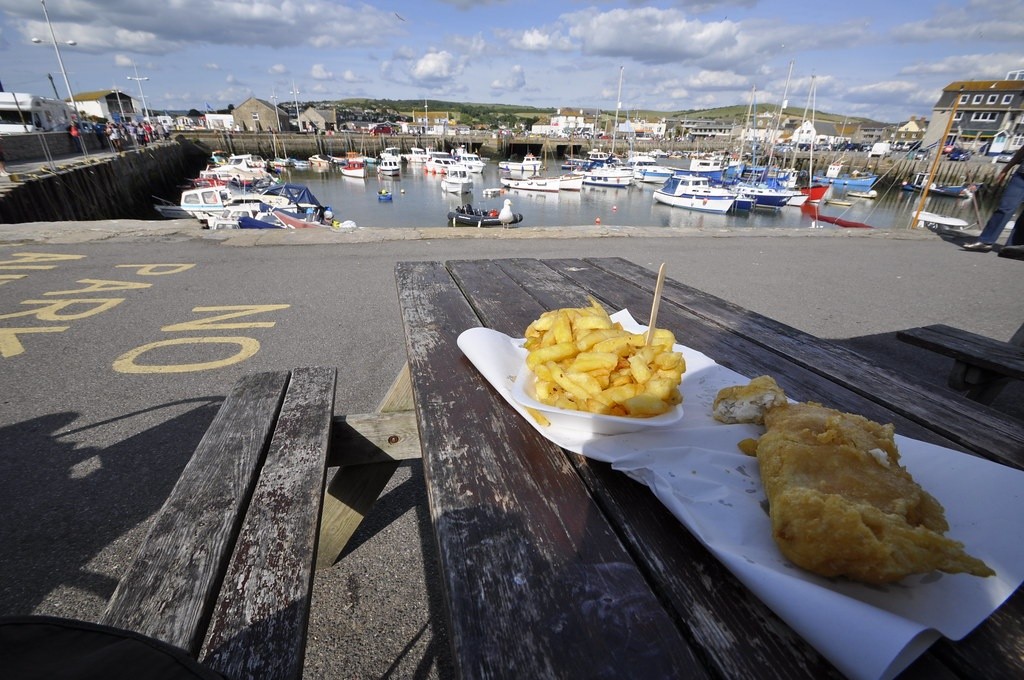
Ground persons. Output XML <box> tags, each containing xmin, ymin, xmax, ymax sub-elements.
<box><xmin>390</xmin><ymin>128</ymin><xmax>397</xmax><ymax>137</ymax></box>
<box><xmin>92</xmin><ymin>122</ymin><xmax>106</xmax><ymax>149</ymax></box>
<box><xmin>105</xmin><ymin>122</ymin><xmax>169</xmax><ymax>152</ymax></box>
<box><xmin>65</xmin><ymin>121</ymin><xmax>83</xmax><ymax>152</ymax></box>
<box><xmin>962</xmin><ymin>145</ymin><xmax>1024</xmax><ymax>252</ymax></box>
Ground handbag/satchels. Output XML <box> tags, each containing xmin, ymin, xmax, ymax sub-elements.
<box><xmin>70</xmin><ymin>127</ymin><xmax>78</xmax><ymax>138</ymax></box>
<box><xmin>144</xmin><ymin>134</ymin><xmax>150</xmax><ymax>143</ymax></box>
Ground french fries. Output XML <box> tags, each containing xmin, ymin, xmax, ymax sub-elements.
<box><xmin>523</xmin><ymin>297</ymin><xmax>687</xmax><ymax>427</ymax></box>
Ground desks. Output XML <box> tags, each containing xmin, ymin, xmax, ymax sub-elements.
<box><xmin>320</xmin><ymin>254</ymin><xmax>1024</xmax><ymax>680</ymax></box>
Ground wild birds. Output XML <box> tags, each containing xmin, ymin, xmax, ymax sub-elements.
<box><xmin>498</xmin><ymin>199</ymin><xmax>514</xmax><ymax>229</ymax></box>
<box><xmin>395</xmin><ymin>13</ymin><xmax>404</xmax><ymax>21</ymax></box>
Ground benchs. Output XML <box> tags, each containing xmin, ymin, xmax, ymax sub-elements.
<box><xmin>896</xmin><ymin>323</ymin><xmax>1024</xmax><ymax>381</ymax></box>
<box><xmin>84</xmin><ymin>366</ymin><xmax>340</xmax><ymax>680</ymax></box>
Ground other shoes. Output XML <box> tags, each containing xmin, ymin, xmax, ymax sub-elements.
<box><xmin>1</xmin><ymin>170</ymin><xmax>12</xmax><ymax>177</ymax></box>
<box><xmin>962</xmin><ymin>242</ymin><xmax>994</xmax><ymax>253</ymax></box>
